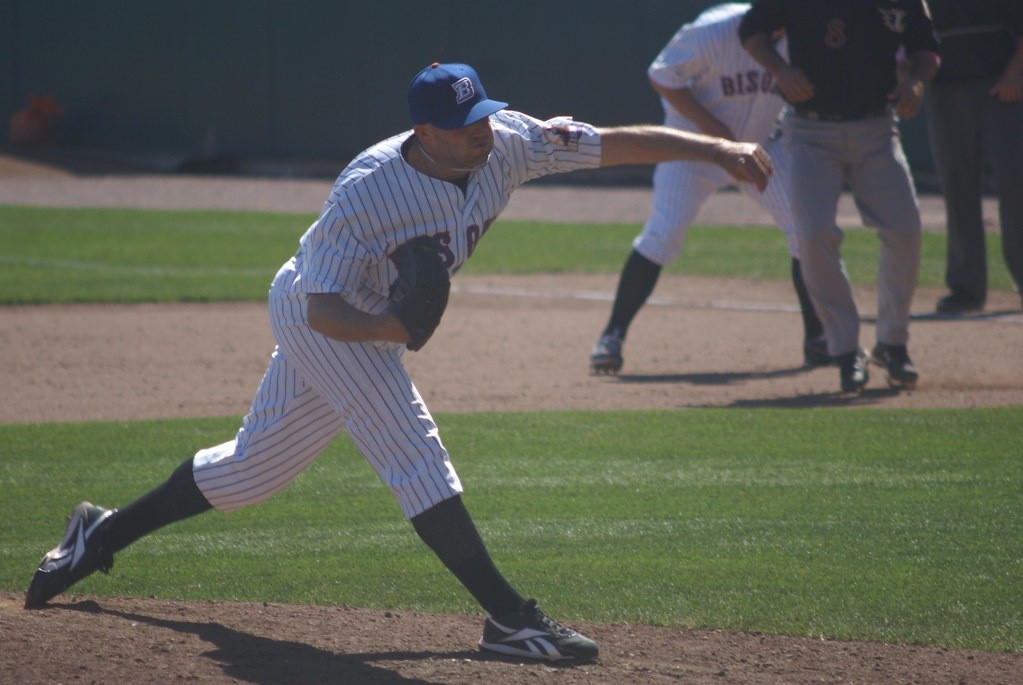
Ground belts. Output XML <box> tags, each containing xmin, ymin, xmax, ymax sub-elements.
<box><xmin>796</xmin><ymin>109</ymin><xmax>889</xmax><ymax>121</ymax></box>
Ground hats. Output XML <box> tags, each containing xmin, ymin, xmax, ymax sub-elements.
<box><xmin>408</xmin><ymin>62</ymin><xmax>509</xmax><ymax>129</ymax></box>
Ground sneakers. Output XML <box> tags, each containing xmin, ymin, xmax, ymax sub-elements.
<box><xmin>837</xmin><ymin>348</ymin><xmax>868</xmax><ymax>390</ymax></box>
<box><xmin>590</xmin><ymin>334</ymin><xmax>623</xmax><ymax>375</ymax></box>
<box><xmin>869</xmin><ymin>343</ymin><xmax>917</xmax><ymax>387</ymax></box>
<box><xmin>803</xmin><ymin>335</ymin><xmax>836</xmax><ymax>365</ymax></box>
<box><xmin>478</xmin><ymin>598</ymin><xmax>599</xmax><ymax>662</ymax></box>
<box><xmin>24</xmin><ymin>501</ymin><xmax>119</xmax><ymax>608</ymax></box>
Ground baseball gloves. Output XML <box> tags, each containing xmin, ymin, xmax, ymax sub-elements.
<box><xmin>383</xmin><ymin>245</ymin><xmax>453</xmax><ymax>354</ymax></box>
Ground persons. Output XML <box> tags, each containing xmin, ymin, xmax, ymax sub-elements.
<box><xmin>897</xmin><ymin>0</ymin><xmax>1023</xmax><ymax>313</ymax></box>
<box><xmin>590</xmin><ymin>1</ymin><xmax>842</xmax><ymax>374</ymax></box>
<box><xmin>737</xmin><ymin>1</ymin><xmax>942</xmax><ymax>394</ymax></box>
<box><xmin>25</xmin><ymin>58</ymin><xmax>774</xmax><ymax>664</ymax></box>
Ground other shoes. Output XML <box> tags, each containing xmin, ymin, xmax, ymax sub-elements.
<box><xmin>935</xmin><ymin>293</ymin><xmax>984</xmax><ymax>314</ymax></box>
